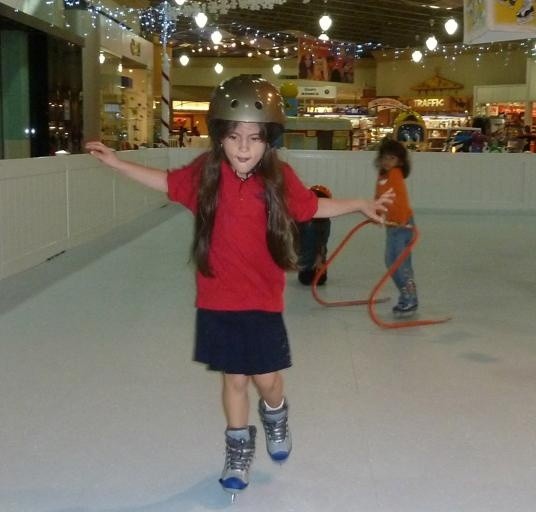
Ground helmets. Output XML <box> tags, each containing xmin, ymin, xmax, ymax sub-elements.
<box><xmin>309</xmin><ymin>184</ymin><xmax>331</xmax><ymax>199</ymax></box>
<box><xmin>205</xmin><ymin>72</ymin><xmax>288</xmax><ymax>129</ymax></box>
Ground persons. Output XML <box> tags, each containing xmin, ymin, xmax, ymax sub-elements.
<box><xmin>299</xmin><ymin>42</ymin><xmax>353</xmax><ymax>84</ymax></box>
<box><xmin>371</xmin><ymin>139</ymin><xmax>419</xmax><ymax>315</ymax></box>
<box><xmin>289</xmin><ymin>185</ymin><xmax>333</xmax><ymax>286</ymax></box>
<box><xmin>190</xmin><ymin>121</ymin><xmax>200</xmax><ymax>136</ymax></box>
<box><xmin>177</xmin><ymin>126</ymin><xmax>189</xmax><ymax>148</ymax></box>
<box><xmin>81</xmin><ymin>72</ymin><xmax>398</xmax><ymax>494</ymax></box>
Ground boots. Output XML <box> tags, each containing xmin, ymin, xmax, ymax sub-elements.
<box><xmin>218</xmin><ymin>423</ymin><xmax>258</xmax><ymax>493</ymax></box>
<box><xmin>257</xmin><ymin>395</ymin><xmax>294</xmax><ymax>462</ymax></box>
<box><xmin>391</xmin><ymin>281</ymin><xmax>420</xmax><ymax>314</ymax></box>
